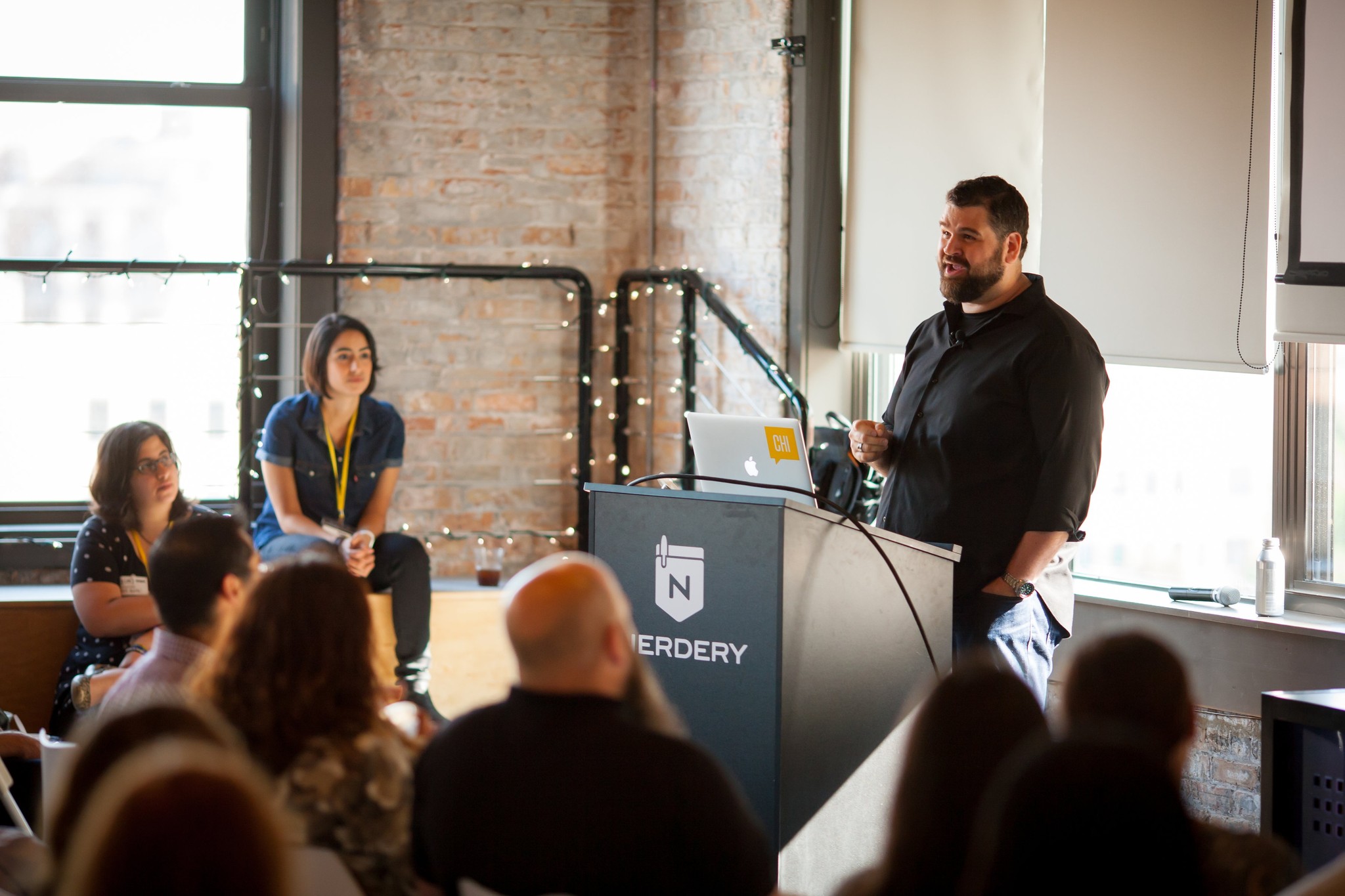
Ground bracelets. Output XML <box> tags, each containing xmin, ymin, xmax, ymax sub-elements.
<box><xmin>125</xmin><ymin>644</ymin><xmax>146</xmax><ymax>655</ymax></box>
<box><xmin>359</xmin><ymin>529</ymin><xmax>375</xmax><ymax>548</ymax></box>
<box><xmin>334</xmin><ymin>535</ymin><xmax>348</xmax><ymax>548</ymax></box>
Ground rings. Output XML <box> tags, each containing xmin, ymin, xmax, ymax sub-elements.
<box><xmin>857</xmin><ymin>442</ymin><xmax>863</xmax><ymax>452</ymax></box>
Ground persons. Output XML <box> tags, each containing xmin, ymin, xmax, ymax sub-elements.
<box><xmin>0</xmin><ymin>422</ymin><xmax>1345</xmax><ymax>896</ymax></box>
<box><xmin>847</xmin><ymin>176</ymin><xmax>1110</xmax><ymax>709</ymax></box>
<box><xmin>252</xmin><ymin>313</ymin><xmax>451</xmax><ymax>726</ymax></box>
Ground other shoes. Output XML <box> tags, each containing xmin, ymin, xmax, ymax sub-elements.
<box><xmin>412</xmin><ymin>691</ymin><xmax>450</xmax><ymax>725</ymax></box>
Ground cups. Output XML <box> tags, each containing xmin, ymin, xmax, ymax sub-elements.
<box><xmin>473</xmin><ymin>547</ymin><xmax>504</xmax><ymax>586</ymax></box>
<box><xmin>379</xmin><ymin>700</ymin><xmax>419</xmax><ymax>739</ymax></box>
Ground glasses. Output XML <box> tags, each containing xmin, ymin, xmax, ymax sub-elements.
<box><xmin>132</xmin><ymin>452</ymin><xmax>176</xmax><ymax>475</ymax></box>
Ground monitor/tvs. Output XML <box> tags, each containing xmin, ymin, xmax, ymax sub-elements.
<box><xmin>684</xmin><ymin>411</ymin><xmax>819</xmax><ymax>510</ymax></box>
<box><xmin>811</xmin><ymin>425</ymin><xmax>861</xmax><ymax>516</ymax></box>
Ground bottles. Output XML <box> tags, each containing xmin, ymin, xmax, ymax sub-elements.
<box><xmin>1255</xmin><ymin>537</ymin><xmax>1285</xmax><ymax>617</ymax></box>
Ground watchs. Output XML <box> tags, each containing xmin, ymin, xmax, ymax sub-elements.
<box><xmin>1001</xmin><ymin>570</ymin><xmax>1034</xmax><ymax>598</ymax></box>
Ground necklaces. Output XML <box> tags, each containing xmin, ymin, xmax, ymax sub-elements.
<box><xmin>138</xmin><ymin>530</ymin><xmax>154</xmax><ymax>545</ymax></box>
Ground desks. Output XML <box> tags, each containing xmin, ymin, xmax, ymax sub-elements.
<box><xmin>0</xmin><ymin>585</ymin><xmax>80</xmax><ymax>733</ymax></box>
<box><xmin>1260</xmin><ymin>688</ymin><xmax>1345</xmax><ymax>896</ymax></box>
